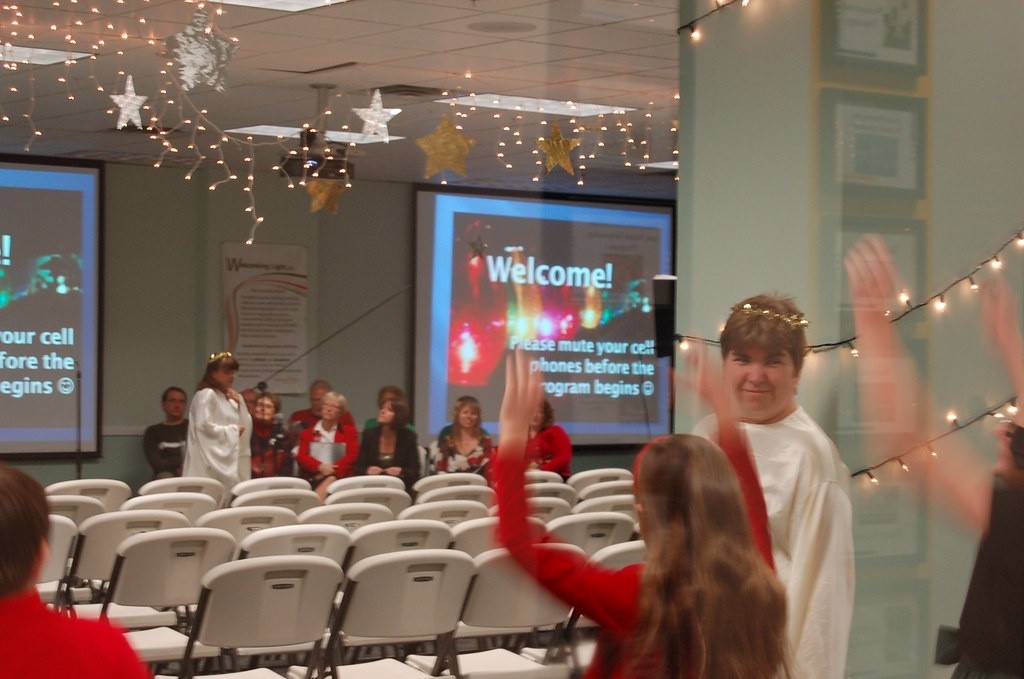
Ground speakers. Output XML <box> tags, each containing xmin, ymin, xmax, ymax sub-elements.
<box><xmin>653</xmin><ymin>274</ymin><xmax>677</xmax><ymax>357</ymax></box>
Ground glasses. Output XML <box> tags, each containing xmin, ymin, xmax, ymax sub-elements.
<box><xmin>310</xmin><ymin>398</ymin><xmax>322</xmax><ymax>404</ymax></box>
<box><xmin>321</xmin><ymin>403</ymin><xmax>339</xmax><ymax>410</ymax></box>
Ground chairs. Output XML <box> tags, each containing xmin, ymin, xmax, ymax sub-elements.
<box><xmin>36</xmin><ymin>468</ymin><xmax>647</xmax><ymax>679</ymax></box>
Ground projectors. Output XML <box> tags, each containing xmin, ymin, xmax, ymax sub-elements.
<box><xmin>279</xmin><ymin>156</ymin><xmax>353</xmax><ymax>182</ymax></box>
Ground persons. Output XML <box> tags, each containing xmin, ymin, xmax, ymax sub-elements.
<box><xmin>0</xmin><ymin>462</ymin><xmax>156</xmax><ymax>679</ymax></box>
<box><xmin>358</xmin><ymin>398</ymin><xmax>421</xmax><ymax>495</ymax></box>
<box><xmin>429</xmin><ymin>396</ymin><xmax>493</xmax><ymax>475</ymax></box>
<box><xmin>242</xmin><ymin>389</ymin><xmax>258</xmax><ymax>416</ymax></box>
<box><xmin>491</xmin><ymin>336</ymin><xmax>793</xmax><ymax>679</ymax></box>
<box><xmin>526</xmin><ymin>398</ymin><xmax>572</xmax><ymax>484</ymax></box>
<box><xmin>143</xmin><ymin>386</ymin><xmax>189</xmax><ymax>481</ymax></box>
<box><xmin>845</xmin><ymin>235</ymin><xmax>1024</xmax><ymax>679</ymax></box>
<box><xmin>183</xmin><ymin>352</ymin><xmax>253</xmax><ymax>504</ymax></box>
<box><xmin>691</xmin><ymin>293</ymin><xmax>854</xmax><ymax>678</ymax></box>
<box><xmin>297</xmin><ymin>391</ymin><xmax>360</xmax><ymax>500</ymax></box>
<box><xmin>287</xmin><ymin>379</ymin><xmax>355</xmax><ymax>434</ymax></box>
<box><xmin>365</xmin><ymin>385</ymin><xmax>415</xmax><ymax>432</ymax></box>
<box><xmin>250</xmin><ymin>393</ymin><xmax>292</xmax><ymax>479</ymax></box>
